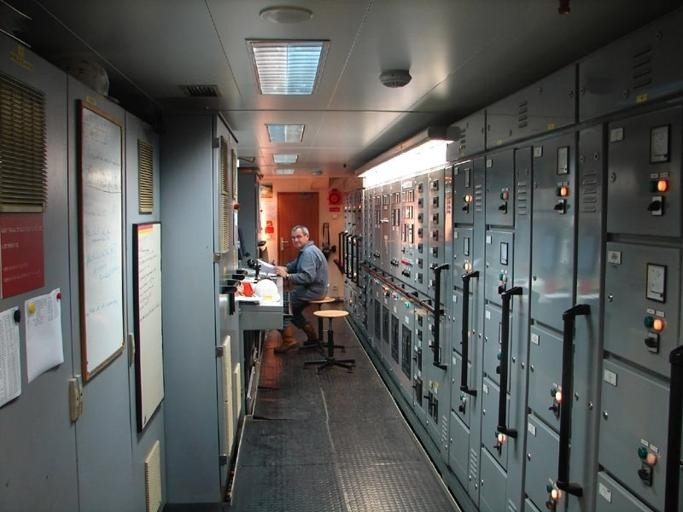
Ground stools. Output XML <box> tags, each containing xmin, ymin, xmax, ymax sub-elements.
<box><xmin>301</xmin><ymin>295</ymin><xmax>346</xmax><ymax>355</ymax></box>
<box><xmin>301</xmin><ymin>309</ymin><xmax>357</xmax><ymax>373</ymax></box>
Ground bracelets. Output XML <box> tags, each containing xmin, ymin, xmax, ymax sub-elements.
<box><xmin>286</xmin><ymin>273</ymin><xmax>288</xmax><ymax>279</ymax></box>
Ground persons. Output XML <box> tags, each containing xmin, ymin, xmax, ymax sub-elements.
<box><xmin>272</xmin><ymin>225</ymin><xmax>328</xmax><ymax>353</ymax></box>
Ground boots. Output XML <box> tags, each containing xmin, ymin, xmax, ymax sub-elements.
<box><xmin>272</xmin><ymin>325</ymin><xmax>299</xmax><ymax>354</ymax></box>
<box><xmin>302</xmin><ymin>321</ymin><xmax>319</xmax><ymax>347</ymax></box>
